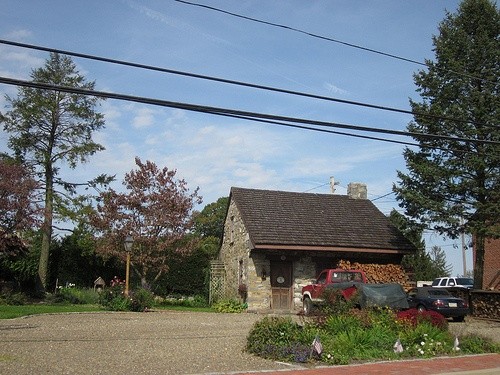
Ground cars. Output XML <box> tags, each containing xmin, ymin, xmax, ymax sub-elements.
<box><xmin>405</xmin><ymin>287</ymin><xmax>469</xmax><ymax>322</ymax></box>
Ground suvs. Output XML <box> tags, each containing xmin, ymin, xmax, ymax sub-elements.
<box><xmin>432</xmin><ymin>277</ymin><xmax>475</xmax><ymax>291</ymax></box>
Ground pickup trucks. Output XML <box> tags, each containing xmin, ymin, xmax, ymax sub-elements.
<box><xmin>301</xmin><ymin>267</ymin><xmax>409</xmax><ymax>316</ymax></box>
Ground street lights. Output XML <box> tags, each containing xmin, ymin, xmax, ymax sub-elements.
<box><xmin>123</xmin><ymin>233</ymin><xmax>134</xmax><ymax>299</ymax></box>
